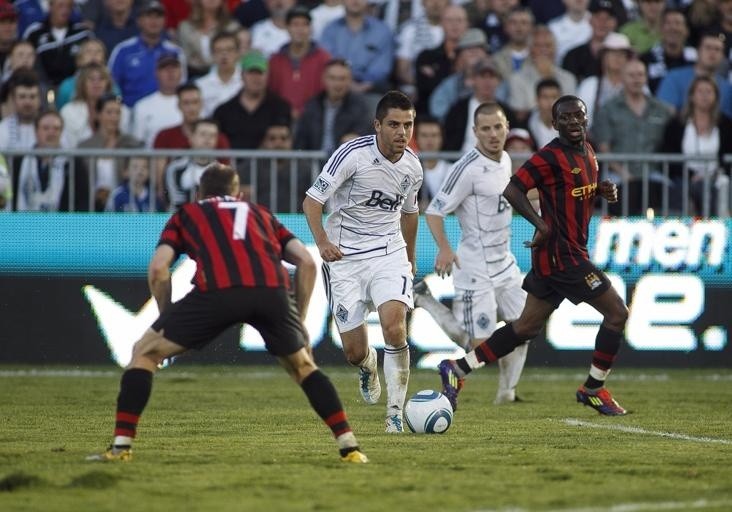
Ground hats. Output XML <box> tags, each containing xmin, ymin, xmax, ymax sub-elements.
<box><xmin>156</xmin><ymin>52</ymin><xmax>180</xmax><ymax>69</ymax></box>
<box><xmin>455</xmin><ymin>27</ymin><xmax>485</xmax><ymax>48</ymax></box>
<box><xmin>603</xmin><ymin>32</ymin><xmax>631</xmax><ymax>51</ymax></box>
<box><xmin>241</xmin><ymin>51</ymin><xmax>266</xmax><ymax>71</ymax></box>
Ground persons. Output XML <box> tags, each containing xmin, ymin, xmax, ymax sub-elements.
<box><xmin>427</xmin><ymin>101</ymin><xmax>529</xmax><ymax>402</ymax></box>
<box><xmin>439</xmin><ymin>96</ymin><xmax>630</xmax><ymax>420</ymax></box>
<box><xmin>301</xmin><ymin>90</ymin><xmax>425</xmax><ymax>435</ymax></box>
<box><xmin>79</xmin><ymin>165</ymin><xmax>369</xmax><ymax>465</ymax></box>
<box><xmin>1</xmin><ymin>1</ymin><xmax>731</xmax><ymax>216</ymax></box>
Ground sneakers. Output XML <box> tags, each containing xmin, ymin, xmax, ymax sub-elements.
<box><xmin>85</xmin><ymin>449</ymin><xmax>132</xmax><ymax>461</ymax></box>
<box><xmin>576</xmin><ymin>385</ymin><xmax>627</xmax><ymax>415</ymax></box>
<box><xmin>438</xmin><ymin>360</ymin><xmax>464</xmax><ymax>412</ymax></box>
<box><xmin>359</xmin><ymin>347</ymin><xmax>381</xmax><ymax>405</ymax></box>
<box><xmin>384</xmin><ymin>414</ymin><xmax>404</xmax><ymax>432</ymax></box>
<box><xmin>341</xmin><ymin>450</ymin><xmax>369</xmax><ymax>464</ymax></box>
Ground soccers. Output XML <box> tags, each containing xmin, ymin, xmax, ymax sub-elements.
<box><xmin>404</xmin><ymin>389</ymin><xmax>454</xmax><ymax>434</ymax></box>
